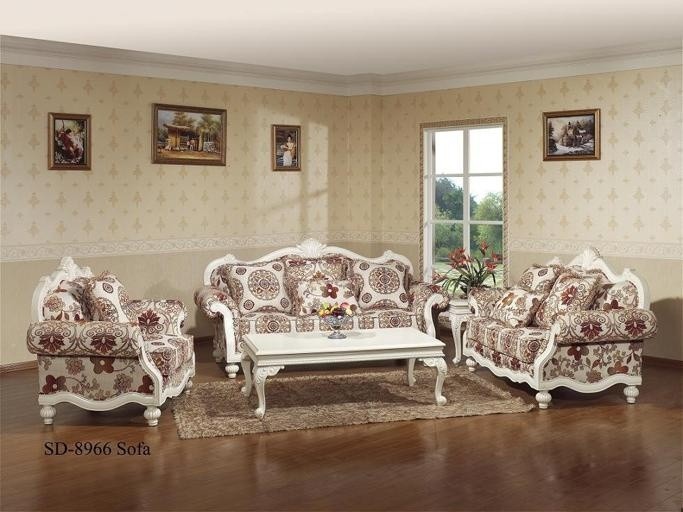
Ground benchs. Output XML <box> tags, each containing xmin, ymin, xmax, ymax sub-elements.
<box><xmin>462</xmin><ymin>245</ymin><xmax>659</xmax><ymax>411</ymax></box>
<box><xmin>193</xmin><ymin>237</ymin><xmax>450</xmax><ymax>378</ymax></box>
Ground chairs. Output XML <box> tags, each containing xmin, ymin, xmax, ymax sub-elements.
<box><xmin>26</xmin><ymin>255</ymin><xmax>197</xmax><ymax>426</ymax></box>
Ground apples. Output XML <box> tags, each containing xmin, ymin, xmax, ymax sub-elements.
<box><xmin>340</xmin><ymin>302</ymin><xmax>350</xmax><ymax>309</ymax></box>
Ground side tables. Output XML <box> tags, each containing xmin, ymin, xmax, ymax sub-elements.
<box><xmin>448</xmin><ymin>297</ymin><xmax>471</xmax><ymax>367</ymax></box>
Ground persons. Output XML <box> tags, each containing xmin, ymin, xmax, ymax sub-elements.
<box><xmin>187</xmin><ymin>135</ymin><xmax>198</xmax><ymax>151</ymax></box>
<box><xmin>281</xmin><ymin>135</ymin><xmax>295</xmax><ymax>167</ymax></box>
<box><xmin>55</xmin><ymin>126</ymin><xmax>83</xmax><ymax>164</ymax></box>
<box><xmin>566</xmin><ymin>120</ymin><xmax>586</xmax><ymax>147</ymax></box>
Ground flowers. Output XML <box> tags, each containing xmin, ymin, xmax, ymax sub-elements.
<box><xmin>431</xmin><ymin>241</ymin><xmax>504</xmax><ymax>296</ymax></box>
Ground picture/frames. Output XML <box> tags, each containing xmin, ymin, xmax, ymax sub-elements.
<box><xmin>271</xmin><ymin>124</ymin><xmax>301</xmax><ymax>172</ymax></box>
<box><xmin>48</xmin><ymin>112</ymin><xmax>91</xmax><ymax>171</ymax></box>
<box><xmin>542</xmin><ymin>107</ymin><xmax>601</xmax><ymax>161</ymax></box>
<box><xmin>151</xmin><ymin>102</ymin><xmax>227</xmax><ymax>166</ymax></box>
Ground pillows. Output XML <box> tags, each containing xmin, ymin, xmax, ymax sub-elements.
<box><xmin>292</xmin><ymin>277</ymin><xmax>363</xmax><ymax>318</ymax></box>
<box><xmin>346</xmin><ymin>259</ymin><xmax>411</xmax><ymax>310</ymax></box>
<box><xmin>533</xmin><ymin>268</ymin><xmax>604</xmax><ymax>329</ymax></box>
<box><xmin>82</xmin><ymin>268</ymin><xmax>139</xmax><ymax>324</ymax></box>
<box><xmin>221</xmin><ymin>259</ymin><xmax>292</xmax><ymax>316</ymax></box>
<box><xmin>489</xmin><ymin>283</ymin><xmax>546</xmax><ymax>329</ymax></box>
<box><xmin>519</xmin><ymin>264</ymin><xmax>566</xmax><ymax>296</ymax></box>
<box><xmin>285</xmin><ymin>255</ymin><xmax>351</xmax><ymax>313</ymax></box>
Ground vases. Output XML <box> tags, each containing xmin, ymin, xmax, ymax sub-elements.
<box><xmin>460</xmin><ymin>285</ymin><xmax>491</xmax><ymax>295</ymax></box>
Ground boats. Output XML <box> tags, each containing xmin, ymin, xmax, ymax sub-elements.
<box><xmin>56</xmin><ymin>131</ymin><xmax>83</xmax><ymax>162</ymax></box>
<box><xmin>559</xmin><ymin>133</ymin><xmax>591</xmax><ymax>148</ymax></box>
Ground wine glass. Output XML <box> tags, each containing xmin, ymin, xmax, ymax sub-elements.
<box><xmin>322</xmin><ymin>315</ymin><xmax>351</xmax><ymax>339</ymax></box>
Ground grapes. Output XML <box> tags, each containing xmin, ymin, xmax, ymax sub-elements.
<box><xmin>332</xmin><ymin>307</ymin><xmax>344</xmax><ymax>317</ymax></box>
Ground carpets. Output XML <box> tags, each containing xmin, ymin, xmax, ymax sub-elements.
<box><xmin>172</xmin><ymin>365</ymin><xmax>536</xmax><ymax>440</ymax></box>
